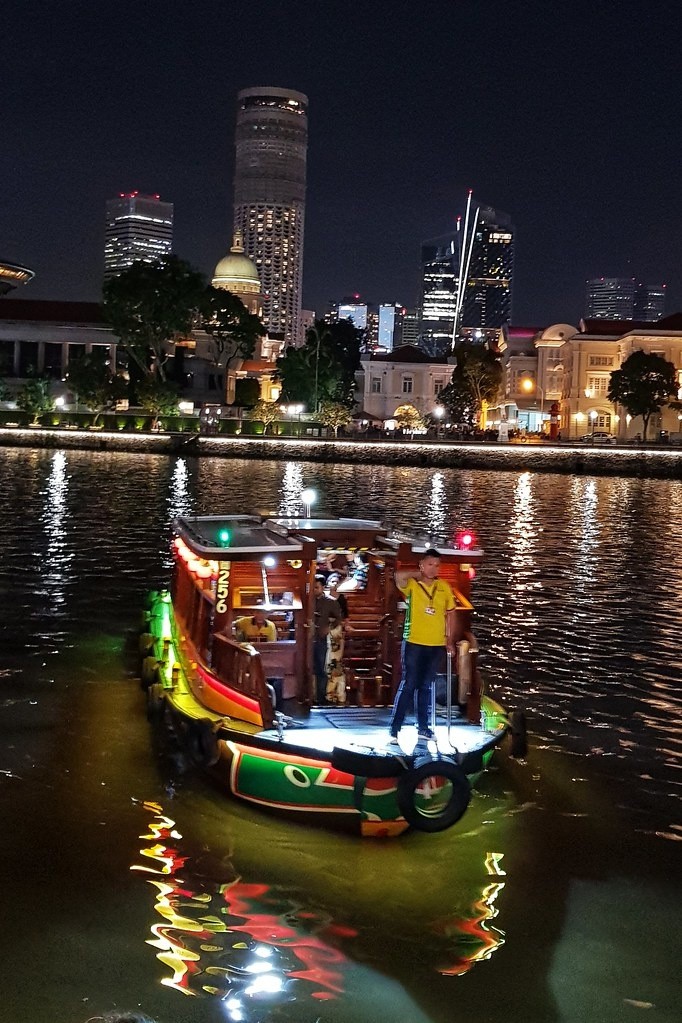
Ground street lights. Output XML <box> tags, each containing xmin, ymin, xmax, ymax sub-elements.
<box><xmin>591</xmin><ymin>411</ymin><xmax>597</xmax><ymax>447</ymax></box>
<box><xmin>523</xmin><ymin>380</ymin><xmax>543</xmax><ymax>436</ymax></box>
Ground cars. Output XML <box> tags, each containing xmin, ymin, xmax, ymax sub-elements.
<box><xmin>581</xmin><ymin>431</ymin><xmax>617</xmax><ymax>445</ymax></box>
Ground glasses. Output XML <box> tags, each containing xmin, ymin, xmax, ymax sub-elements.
<box><xmin>332</xmin><ymin>581</ymin><xmax>338</xmax><ymax>584</ymax></box>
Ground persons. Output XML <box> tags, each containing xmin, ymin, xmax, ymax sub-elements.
<box><xmin>323</xmin><ymin>573</ymin><xmax>348</xmax><ymax>705</ymax></box>
<box><xmin>234</xmin><ymin>609</ymin><xmax>278</xmax><ymax>644</ymax></box>
<box><xmin>389</xmin><ymin>549</ymin><xmax>457</xmax><ymax>744</ymax></box>
<box><xmin>312</xmin><ymin>574</ymin><xmax>343</xmax><ymax>706</ymax></box>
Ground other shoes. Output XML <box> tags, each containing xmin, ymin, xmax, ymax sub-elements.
<box><xmin>418</xmin><ymin>729</ymin><xmax>434</xmax><ymax>740</ymax></box>
<box><xmin>390</xmin><ymin>737</ymin><xmax>398</xmax><ymax>744</ymax></box>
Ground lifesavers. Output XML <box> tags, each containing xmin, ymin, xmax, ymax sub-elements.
<box><xmin>136</xmin><ymin>591</ymin><xmax>222</xmax><ymax>769</ymax></box>
<box><xmin>507</xmin><ymin>708</ymin><xmax>530</xmax><ymax>760</ymax></box>
<box><xmin>395</xmin><ymin>753</ymin><xmax>473</xmax><ymax>834</ymax></box>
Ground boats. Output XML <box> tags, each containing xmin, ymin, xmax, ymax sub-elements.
<box><xmin>140</xmin><ymin>489</ymin><xmax>529</xmax><ymax>838</ymax></box>
